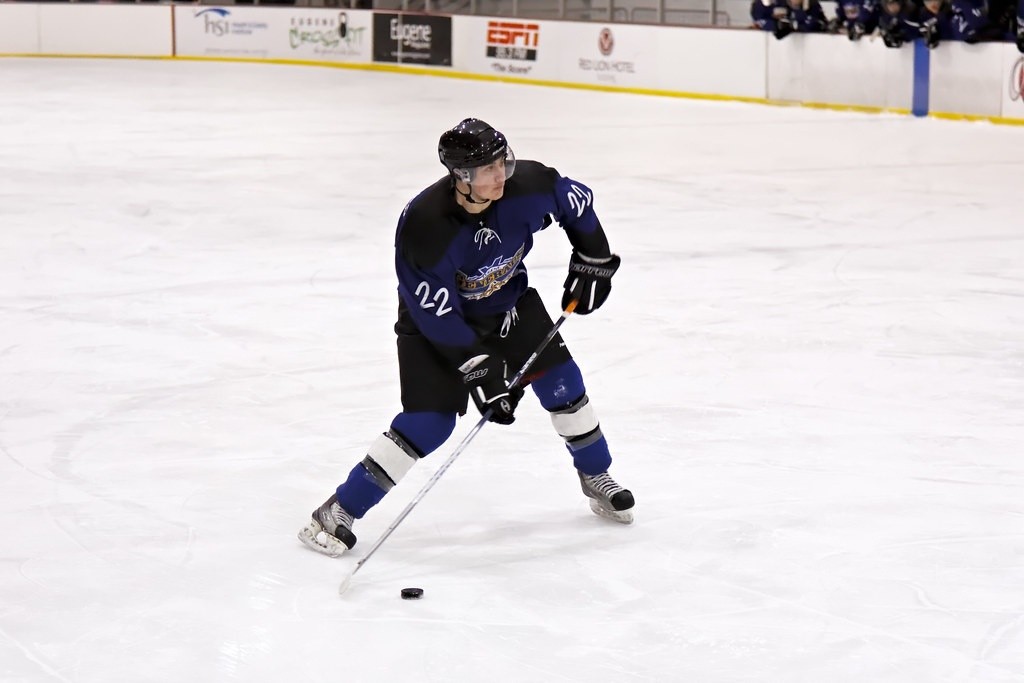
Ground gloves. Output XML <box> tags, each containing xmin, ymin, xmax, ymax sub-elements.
<box><xmin>561</xmin><ymin>250</ymin><xmax>621</xmax><ymax>316</ymax></box>
<box><xmin>461</xmin><ymin>355</ymin><xmax>525</xmax><ymax>424</ymax></box>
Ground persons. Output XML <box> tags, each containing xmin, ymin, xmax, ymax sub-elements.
<box><xmin>749</xmin><ymin>0</ymin><xmax>1024</xmax><ymax>54</ymax></box>
<box><xmin>296</xmin><ymin>118</ymin><xmax>635</xmax><ymax>558</ymax></box>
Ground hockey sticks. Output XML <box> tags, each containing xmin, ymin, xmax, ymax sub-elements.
<box><xmin>336</xmin><ymin>300</ymin><xmax>577</xmax><ymax>597</ymax></box>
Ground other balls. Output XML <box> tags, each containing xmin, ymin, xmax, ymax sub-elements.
<box><xmin>401</xmin><ymin>587</ymin><xmax>424</xmax><ymax>600</ymax></box>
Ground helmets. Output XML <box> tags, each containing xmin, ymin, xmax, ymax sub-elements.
<box><xmin>437</xmin><ymin>118</ymin><xmax>517</xmax><ymax>206</ymax></box>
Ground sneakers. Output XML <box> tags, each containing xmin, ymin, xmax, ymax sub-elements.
<box><xmin>576</xmin><ymin>473</ymin><xmax>635</xmax><ymax>525</ymax></box>
<box><xmin>297</xmin><ymin>494</ymin><xmax>357</xmax><ymax>557</ymax></box>
